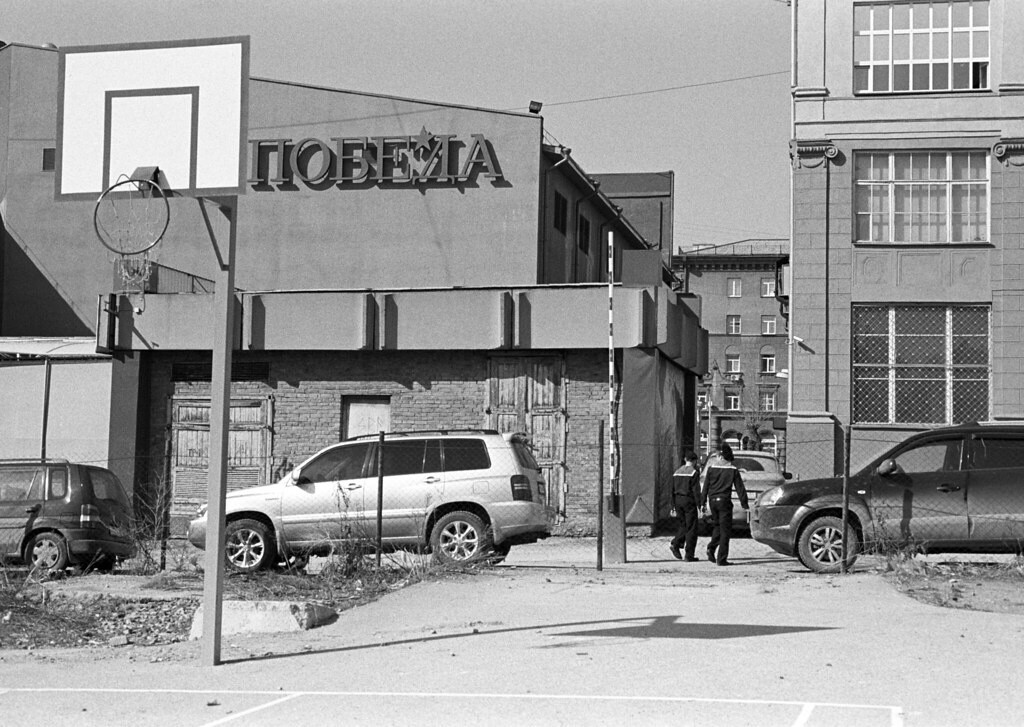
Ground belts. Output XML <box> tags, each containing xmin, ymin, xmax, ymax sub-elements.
<box><xmin>711</xmin><ymin>497</ymin><xmax>724</xmax><ymax>501</ymax></box>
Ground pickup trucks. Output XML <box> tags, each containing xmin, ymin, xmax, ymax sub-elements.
<box><xmin>698</xmin><ymin>450</ymin><xmax>793</xmax><ymax>531</ymax></box>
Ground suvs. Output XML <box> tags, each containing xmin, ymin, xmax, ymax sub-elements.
<box><xmin>186</xmin><ymin>426</ymin><xmax>550</xmax><ymax>575</ymax></box>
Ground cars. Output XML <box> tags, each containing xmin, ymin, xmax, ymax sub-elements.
<box><xmin>0</xmin><ymin>455</ymin><xmax>137</xmax><ymax>580</ymax></box>
<box><xmin>747</xmin><ymin>418</ymin><xmax>1024</xmax><ymax>576</ymax></box>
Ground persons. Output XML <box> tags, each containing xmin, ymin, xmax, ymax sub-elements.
<box><xmin>670</xmin><ymin>450</ymin><xmax>701</xmax><ymax>562</ymax></box>
<box><xmin>701</xmin><ymin>442</ymin><xmax>749</xmax><ymax>566</ymax></box>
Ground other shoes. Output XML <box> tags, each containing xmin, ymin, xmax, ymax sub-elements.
<box><xmin>670</xmin><ymin>543</ymin><xmax>682</xmax><ymax>559</ymax></box>
<box><xmin>707</xmin><ymin>549</ymin><xmax>716</xmax><ymax>563</ymax></box>
<box><xmin>685</xmin><ymin>556</ymin><xmax>699</xmax><ymax>562</ymax></box>
<box><xmin>717</xmin><ymin>561</ymin><xmax>734</xmax><ymax>566</ymax></box>
